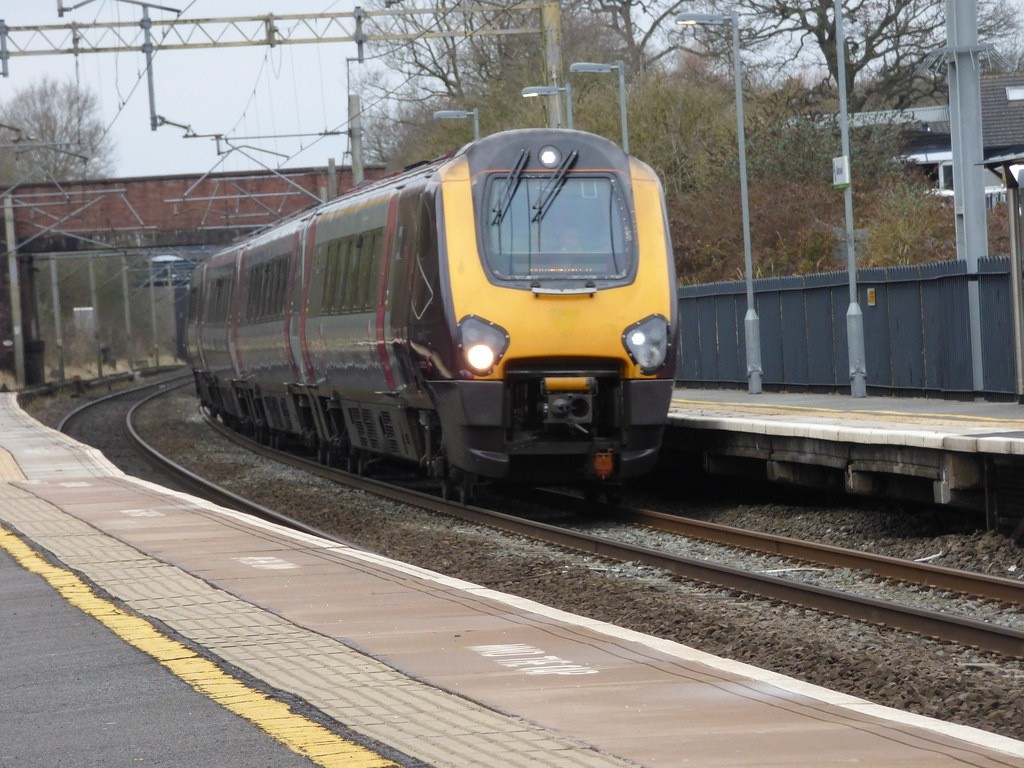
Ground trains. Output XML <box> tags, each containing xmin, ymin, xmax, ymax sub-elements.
<box><xmin>181</xmin><ymin>127</ymin><xmax>681</xmax><ymax>510</ymax></box>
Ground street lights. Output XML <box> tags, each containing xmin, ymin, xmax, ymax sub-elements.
<box><xmin>674</xmin><ymin>11</ymin><xmax>766</xmax><ymax>396</ymax></box>
<box><xmin>569</xmin><ymin>58</ymin><xmax>628</xmax><ymax>154</ymax></box>
<box><xmin>521</xmin><ymin>81</ymin><xmax>574</xmax><ymax>129</ymax></box>
<box><xmin>433</xmin><ymin>107</ymin><xmax>481</xmax><ymax>142</ymax></box>
<box><xmin>834</xmin><ymin>0</ymin><xmax>868</xmax><ymax>398</ymax></box>
